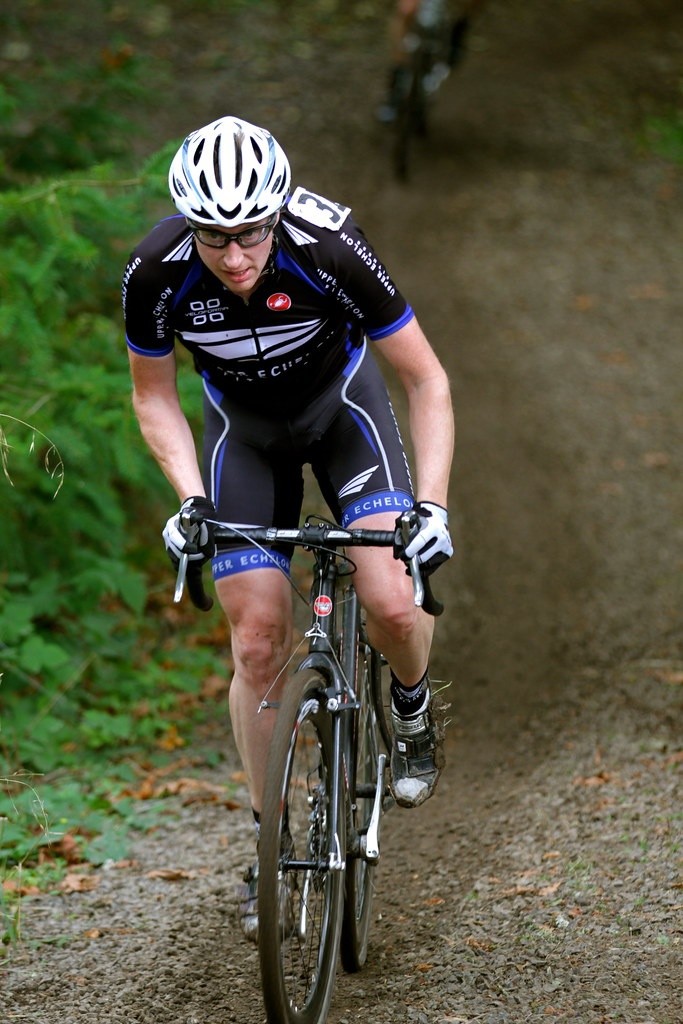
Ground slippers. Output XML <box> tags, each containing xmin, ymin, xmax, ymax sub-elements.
<box><xmin>186</xmin><ymin>212</ymin><xmax>279</xmax><ymax>248</ymax></box>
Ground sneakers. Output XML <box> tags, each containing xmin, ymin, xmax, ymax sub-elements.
<box><xmin>239</xmin><ymin>848</ymin><xmax>300</xmax><ymax>943</ymax></box>
<box><xmin>390</xmin><ymin>690</ymin><xmax>443</xmax><ymax>807</ymax></box>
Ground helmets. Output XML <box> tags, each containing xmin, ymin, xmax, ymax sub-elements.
<box><xmin>167</xmin><ymin>116</ymin><xmax>290</xmax><ymax>227</ymax></box>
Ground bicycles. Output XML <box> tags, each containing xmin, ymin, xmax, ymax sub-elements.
<box><xmin>171</xmin><ymin>496</ymin><xmax>451</xmax><ymax>1024</ymax></box>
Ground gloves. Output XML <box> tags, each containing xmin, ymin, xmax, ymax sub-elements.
<box><xmin>393</xmin><ymin>501</ymin><xmax>456</xmax><ymax>577</ymax></box>
<box><xmin>162</xmin><ymin>496</ymin><xmax>219</xmax><ymax>574</ymax></box>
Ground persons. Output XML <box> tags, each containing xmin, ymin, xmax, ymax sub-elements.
<box><xmin>123</xmin><ymin>114</ymin><xmax>456</xmax><ymax>941</ymax></box>
